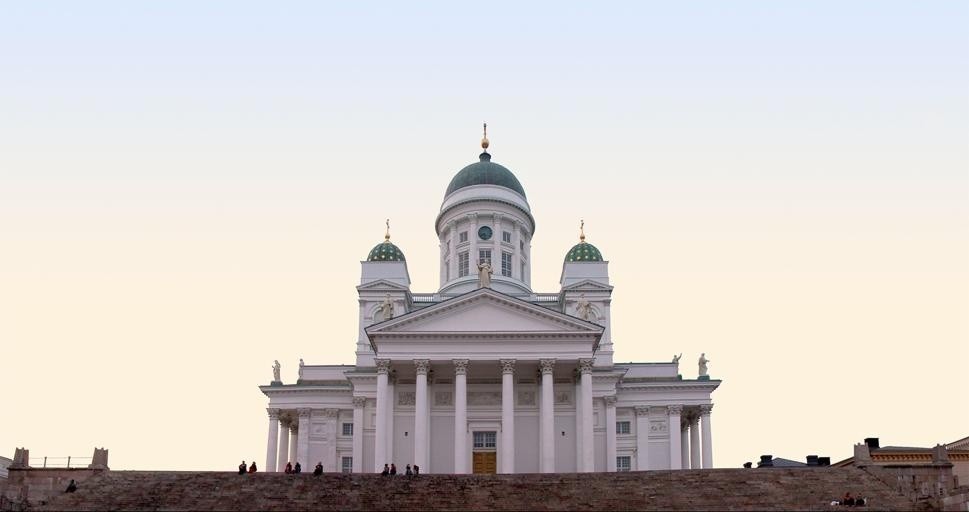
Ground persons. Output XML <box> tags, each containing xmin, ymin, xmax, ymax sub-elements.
<box><xmin>477</xmin><ymin>257</ymin><xmax>493</xmax><ymax>288</ymax></box>
<box><xmin>382</xmin><ymin>464</ymin><xmax>389</xmax><ymax>475</ymax></box>
<box><xmin>297</xmin><ymin>358</ymin><xmax>304</xmax><ymax>377</ymax></box>
<box><xmin>285</xmin><ymin>462</ymin><xmax>291</xmax><ymax>473</ymax></box>
<box><xmin>381</xmin><ymin>291</ymin><xmax>394</xmax><ymax>319</ymax></box>
<box><xmin>855</xmin><ymin>492</ymin><xmax>865</xmax><ymax>504</ymax></box>
<box><xmin>65</xmin><ymin>478</ymin><xmax>76</xmax><ymax>494</ymax></box>
<box><xmin>390</xmin><ymin>464</ymin><xmax>397</xmax><ymax>474</ymax></box>
<box><xmin>239</xmin><ymin>461</ymin><xmax>246</xmax><ymax>475</ymax></box>
<box><xmin>250</xmin><ymin>462</ymin><xmax>256</xmax><ymax>473</ymax></box>
<box><xmin>406</xmin><ymin>464</ymin><xmax>412</xmax><ymax>474</ymax></box>
<box><xmin>844</xmin><ymin>492</ymin><xmax>854</xmax><ymax>505</ymax></box>
<box><xmin>294</xmin><ymin>462</ymin><xmax>301</xmax><ymax>473</ymax></box>
<box><xmin>576</xmin><ymin>293</ymin><xmax>593</xmax><ymax>321</ymax></box>
<box><xmin>313</xmin><ymin>462</ymin><xmax>323</xmax><ymax>475</ymax></box>
<box><xmin>413</xmin><ymin>464</ymin><xmax>419</xmax><ymax>475</ymax></box>
<box><xmin>699</xmin><ymin>353</ymin><xmax>709</xmax><ymax>374</ymax></box>
<box><xmin>272</xmin><ymin>360</ymin><xmax>280</xmax><ymax>380</ymax></box>
<box><xmin>672</xmin><ymin>353</ymin><xmax>681</xmax><ymax>363</ymax></box>
